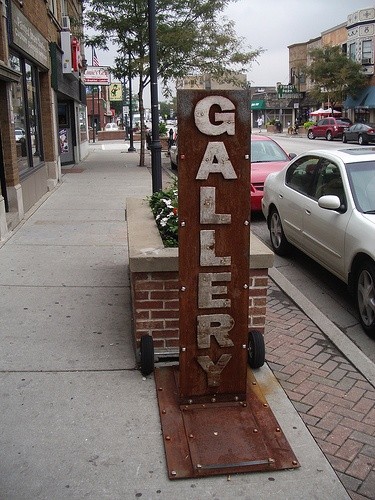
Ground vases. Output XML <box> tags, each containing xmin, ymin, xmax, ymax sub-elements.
<box><xmin>125</xmin><ymin>196</ymin><xmax>274</xmax><ymax>354</ymax></box>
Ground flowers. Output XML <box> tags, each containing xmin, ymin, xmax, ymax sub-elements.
<box><xmin>143</xmin><ymin>175</ymin><xmax>179</xmax><ymax>247</ymax></box>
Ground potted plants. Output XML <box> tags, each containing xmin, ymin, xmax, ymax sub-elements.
<box><xmin>158</xmin><ymin>121</ymin><xmax>168</xmax><ymax>151</ymax></box>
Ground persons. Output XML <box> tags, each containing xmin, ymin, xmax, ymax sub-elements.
<box><xmin>288</xmin><ymin>118</ymin><xmax>298</xmax><ymax>134</ymax></box>
<box><xmin>255</xmin><ymin>116</ymin><xmax>263</xmax><ymax>133</ymax></box>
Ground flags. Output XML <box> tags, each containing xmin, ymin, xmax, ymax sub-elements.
<box><xmin>93</xmin><ymin>48</ymin><xmax>99</xmax><ymax>66</ymax></box>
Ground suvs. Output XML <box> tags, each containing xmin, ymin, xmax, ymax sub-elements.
<box><xmin>307</xmin><ymin>117</ymin><xmax>353</xmax><ymax>141</ymax></box>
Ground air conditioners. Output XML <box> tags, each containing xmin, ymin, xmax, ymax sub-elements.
<box><xmin>61</xmin><ymin>15</ymin><xmax>71</xmax><ymax>30</ymax></box>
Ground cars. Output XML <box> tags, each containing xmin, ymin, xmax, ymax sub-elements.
<box><xmin>14</xmin><ymin>129</ymin><xmax>26</xmax><ymax>143</ymax></box>
<box><xmin>169</xmin><ymin>133</ymin><xmax>178</xmax><ymax>170</ymax></box>
<box><xmin>126</xmin><ymin>114</ymin><xmax>177</xmax><ymax>142</ymax></box>
<box><xmin>250</xmin><ymin>133</ymin><xmax>298</xmax><ymax>212</ymax></box>
<box><xmin>261</xmin><ymin>146</ymin><xmax>375</xmax><ymax>341</ymax></box>
<box><xmin>342</xmin><ymin>122</ymin><xmax>375</xmax><ymax>145</ymax></box>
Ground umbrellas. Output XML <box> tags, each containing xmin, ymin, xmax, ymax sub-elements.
<box><xmin>309</xmin><ymin>108</ymin><xmax>342</xmax><ymax>120</ymax></box>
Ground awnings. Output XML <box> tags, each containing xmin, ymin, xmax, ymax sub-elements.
<box><xmin>344</xmin><ymin>85</ymin><xmax>375</xmax><ymax>109</ymax></box>
<box><xmin>251</xmin><ymin>99</ymin><xmax>265</xmax><ymax>111</ymax></box>
